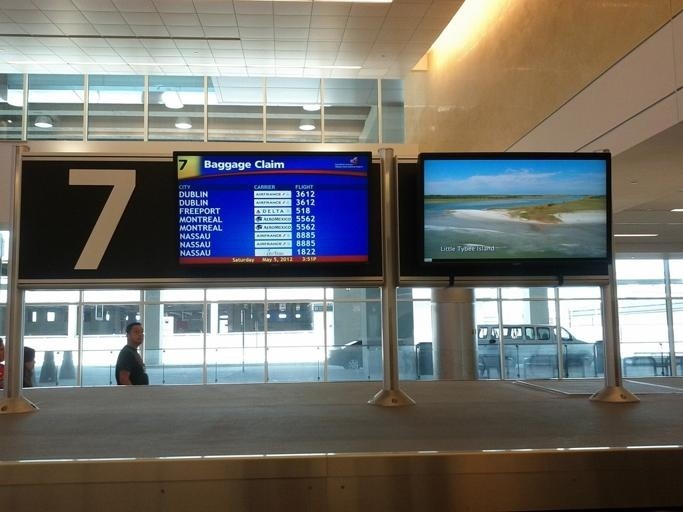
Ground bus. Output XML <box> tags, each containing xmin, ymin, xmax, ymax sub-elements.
<box><xmin>476</xmin><ymin>324</ymin><xmax>595</xmax><ymax>372</ymax></box>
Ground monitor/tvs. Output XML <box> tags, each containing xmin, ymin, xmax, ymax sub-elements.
<box><xmin>173</xmin><ymin>150</ymin><xmax>374</xmax><ymax>278</ymax></box>
<box><xmin>416</xmin><ymin>151</ymin><xmax>614</xmax><ymax>271</ymax></box>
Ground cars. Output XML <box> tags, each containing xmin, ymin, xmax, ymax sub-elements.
<box><xmin>327</xmin><ymin>340</ymin><xmax>363</xmax><ymax>372</ymax></box>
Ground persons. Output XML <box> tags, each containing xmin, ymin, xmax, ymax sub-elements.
<box><xmin>0</xmin><ymin>338</ymin><xmax>4</xmax><ymax>389</ymax></box>
<box><xmin>115</xmin><ymin>322</ymin><xmax>149</xmax><ymax>385</ymax></box>
<box><xmin>23</xmin><ymin>346</ymin><xmax>36</xmax><ymax>388</ymax></box>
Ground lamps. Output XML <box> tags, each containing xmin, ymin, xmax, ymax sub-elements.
<box><xmin>34</xmin><ymin>116</ymin><xmax>53</xmax><ymax>129</ymax></box>
<box><xmin>174</xmin><ymin>116</ymin><xmax>193</xmax><ymax>129</ymax></box>
<box><xmin>299</xmin><ymin>119</ymin><xmax>316</xmax><ymax>131</ymax></box>
